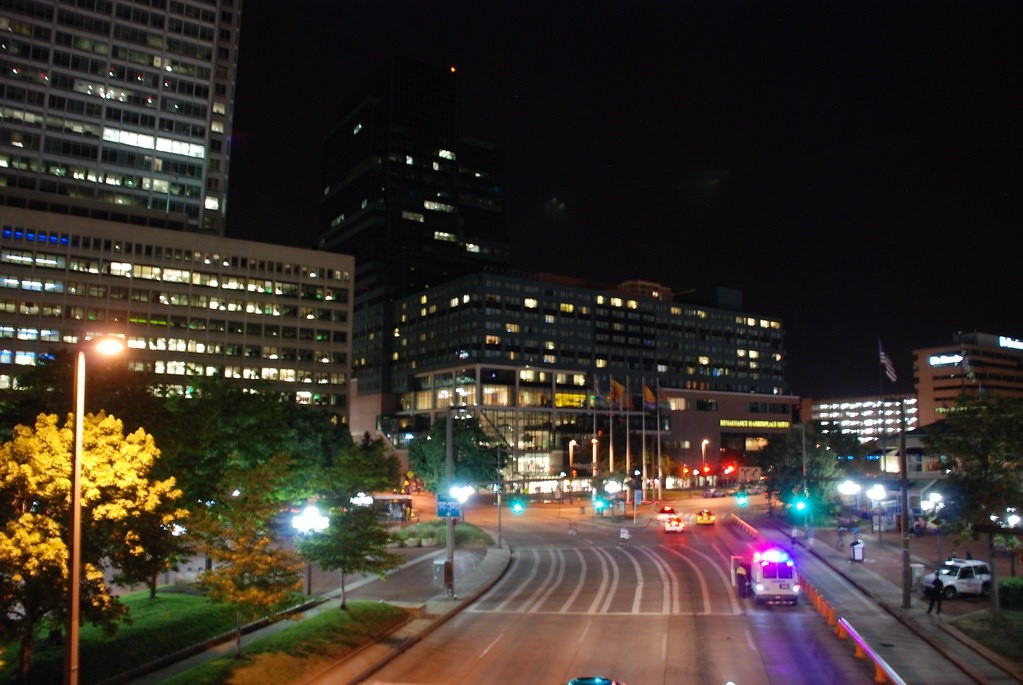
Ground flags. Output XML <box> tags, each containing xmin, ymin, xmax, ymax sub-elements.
<box><xmin>610</xmin><ymin>380</ymin><xmax>632</xmax><ymax>410</ymax></box>
<box><xmin>658</xmin><ymin>386</ymin><xmax>672</xmax><ymax>416</ymax></box>
<box><xmin>643</xmin><ymin>384</ymin><xmax>657</xmax><ymax>416</ymax></box>
<box><xmin>880</xmin><ymin>344</ymin><xmax>897</xmax><ymax>382</ymax></box>
<box><xmin>595</xmin><ymin>380</ymin><xmax>606</xmax><ymax>405</ymax></box>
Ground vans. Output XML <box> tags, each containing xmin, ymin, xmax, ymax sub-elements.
<box><xmin>731</xmin><ymin>550</ymin><xmax>802</xmax><ymax>606</ymax></box>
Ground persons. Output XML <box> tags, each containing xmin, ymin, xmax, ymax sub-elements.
<box><xmin>791</xmin><ymin>525</ymin><xmax>815</xmax><ymax>549</ymax></box>
<box><xmin>909</xmin><ymin>518</ymin><xmax>925</xmax><ymax>537</ymax></box>
<box><xmin>926</xmin><ymin>573</ymin><xmax>943</xmax><ymax>613</ymax></box>
<box><xmin>966</xmin><ymin>551</ymin><xmax>974</xmax><ymax>560</ymax></box>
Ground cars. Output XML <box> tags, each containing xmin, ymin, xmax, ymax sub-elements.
<box><xmin>728</xmin><ymin>484</ymin><xmax>778</xmax><ymax>499</ymax></box>
<box><xmin>656</xmin><ymin>505</ymin><xmax>678</xmax><ymax>522</ymax></box>
<box><xmin>663</xmin><ymin>517</ymin><xmax>684</xmax><ymax>533</ymax></box>
<box><xmin>697</xmin><ymin>510</ymin><xmax>715</xmax><ymax>526</ymax></box>
<box><xmin>704</xmin><ymin>488</ymin><xmax>726</xmax><ymax>498</ymax></box>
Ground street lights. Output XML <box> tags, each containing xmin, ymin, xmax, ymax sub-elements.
<box><xmin>61</xmin><ymin>336</ymin><xmax>124</xmax><ymax>685</ymax></box>
<box><xmin>866</xmin><ymin>482</ymin><xmax>886</xmax><ymax>543</ymax></box>
<box><xmin>1008</xmin><ymin>513</ymin><xmax>1020</xmax><ymax>577</ymax></box>
<box><xmin>700</xmin><ymin>438</ymin><xmax>711</xmax><ymax>466</ymax></box>
<box><xmin>451</xmin><ymin>483</ymin><xmax>476</xmax><ymax>523</ymax></box>
<box><xmin>292</xmin><ymin>504</ymin><xmax>331</xmax><ymax>595</ymax></box>
<box><xmin>837</xmin><ymin>479</ymin><xmax>862</xmax><ymax>529</ymax></box>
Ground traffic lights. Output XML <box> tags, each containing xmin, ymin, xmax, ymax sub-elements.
<box><xmin>736</xmin><ymin>487</ymin><xmax>747</xmax><ymax>508</ymax></box>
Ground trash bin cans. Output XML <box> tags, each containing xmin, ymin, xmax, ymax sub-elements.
<box><xmin>908</xmin><ymin>563</ymin><xmax>925</xmax><ymax>591</ymax></box>
<box><xmin>848</xmin><ymin>539</ymin><xmax>865</xmax><ymax>561</ymax></box>
<box><xmin>580</xmin><ymin>506</ymin><xmax>585</xmax><ymax>515</ymax></box>
<box><xmin>434</xmin><ymin>560</ymin><xmax>452</xmax><ymax>587</ymax></box>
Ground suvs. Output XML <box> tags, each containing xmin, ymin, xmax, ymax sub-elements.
<box><xmin>923</xmin><ymin>557</ymin><xmax>992</xmax><ymax>598</ymax></box>
